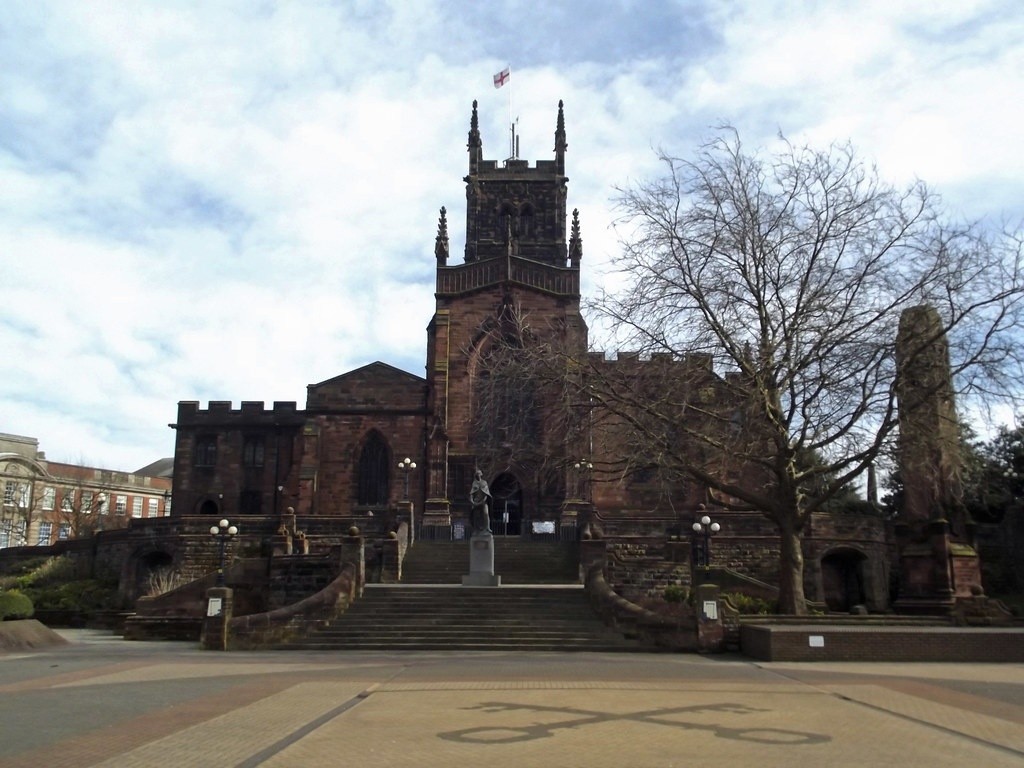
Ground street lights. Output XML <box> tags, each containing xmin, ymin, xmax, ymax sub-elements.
<box><xmin>97</xmin><ymin>492</ymin><xmax>105</xmax><ymax>534</ymax></box>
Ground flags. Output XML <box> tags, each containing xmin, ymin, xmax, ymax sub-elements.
<box><xmin>493</xmin><ymin>67</ymin><xmax>510</xmax><ymax>89</ymax></box>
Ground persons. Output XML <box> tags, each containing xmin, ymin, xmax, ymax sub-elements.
<box><xmin>469</xmin><ymin>470</ymin><xmax>492</xmax><ymax>534</ymax></box>
